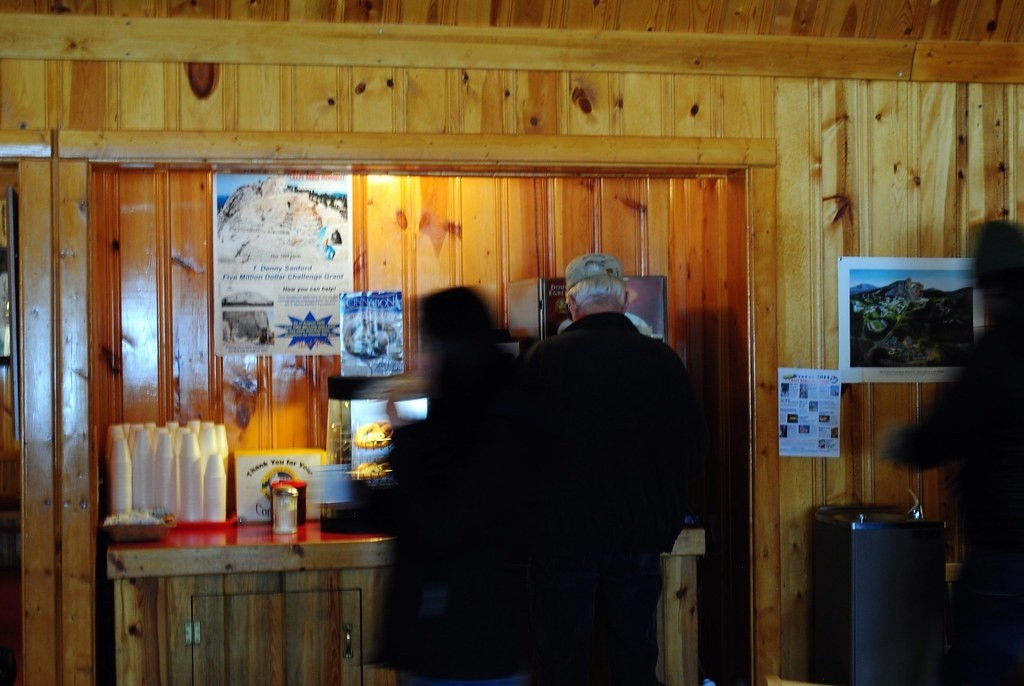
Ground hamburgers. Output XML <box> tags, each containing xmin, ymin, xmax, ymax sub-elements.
<box><xmin>351</xmin><ymin>462</ymin><xmax>390</xmax><ymax>479</ymax></box>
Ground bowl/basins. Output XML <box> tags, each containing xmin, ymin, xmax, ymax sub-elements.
<box><xmin>103</xmin><ymin>517</ymin><xmax>171</xmax><ymax>542</ymax></box>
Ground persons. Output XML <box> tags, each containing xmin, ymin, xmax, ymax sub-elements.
<box><xmin>369</xmin><ymin>288</ymin><xmax>581</xmax><ymax>686</ymax></box>
<box><xmin>885</xmin><ymin>221</ymin><xmax>1024</xmax><ymax>686</ymax></box>
<box><xmin>524</xmin><ymin>254</ymin><xmax>699</xmax><ymax>686</ymax></box>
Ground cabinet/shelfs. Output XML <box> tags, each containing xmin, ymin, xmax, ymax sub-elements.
<box><xmin>106</xmin><ymin>524</ymin><xmax>705</xmax><ymax>686</ymax></box>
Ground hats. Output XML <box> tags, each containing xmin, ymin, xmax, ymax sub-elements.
<box><xmin>565</xmin><ymin>253</ymin><xmax>623</xmax><ymax>291</ymax></box>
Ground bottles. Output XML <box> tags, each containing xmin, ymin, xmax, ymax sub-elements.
<box><xmin>271</xmin><ymin>485</ymin><xmax>299</xmax><ymax>534</ymax></box>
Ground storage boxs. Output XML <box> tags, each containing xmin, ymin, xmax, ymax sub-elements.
<box><xmin>508</xmin><ymin>275</ymin><xmax>668</xmax><ymax>345</ymax></box>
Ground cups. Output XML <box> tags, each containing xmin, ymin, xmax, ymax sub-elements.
<box><xmin>314</xmin><ymin>463</ymin><xmax>353</xmax><ymax>504</ymax></box>
<box><xmin>103</xmin><ymin>418</ymin><xmax>231</xmax><ymax>522</ymax></box>
<box><xmin>319</xmin><ymin>503</ymin><xmax>351</xmax><ymax>532</ymax></box>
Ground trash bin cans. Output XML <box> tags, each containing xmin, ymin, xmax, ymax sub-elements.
<box><xmin>810</xmin><ymin>505</ymin><xmax>947</xmax><ymax>686</ymax></box>
